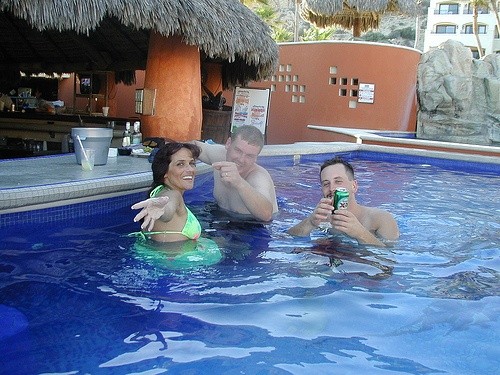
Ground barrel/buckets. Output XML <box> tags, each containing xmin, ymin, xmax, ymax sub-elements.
<box><xmin>71</xmin><ymin>128</ymin><xmax>114</xmax><ymax>166</ymax></box>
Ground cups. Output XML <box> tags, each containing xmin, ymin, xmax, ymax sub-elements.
<box><xmin>80</xmin><ymin>148</ymin><xmax>96</xmax><ymax>170</ymax></box>
<box><xmin>102</xmin><ymin>107</ymin><xmax>109</xmax><ymax>116</ymax></box>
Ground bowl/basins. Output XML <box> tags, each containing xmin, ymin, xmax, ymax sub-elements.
<box><xmin>119</xmin><ymin>148</ymin><xmax>132</xmax><ymax>155</ymax></box>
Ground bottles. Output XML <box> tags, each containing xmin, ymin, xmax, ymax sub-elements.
<box><xmin>135</xmin><ymin>121</ymin><xmax>143</xmax><ymax>142</ymax></box>
<box><xmin>133</xmin><ymin>123</ymin><xmax>141</xmax><ymax>144</ymax></box>
<box><xmin>122</xmin><ymin>131</ymin><xmax>130</xmax><ymax>147</ymax></box>
<box><xmin>123</xmin><ymin>122</ymin><xmax>132</xmax><ymax>144</ymax></box>
<box><xmin>23</xmin><ymin>137</ymin><xmax>47</xmax><ymax>151</ymax></box>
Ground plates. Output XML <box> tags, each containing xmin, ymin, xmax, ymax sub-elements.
<box><xmin>91</xmin><ymin>113</ymin><xmax>102</xmax><ymax>115</ymax></box>
<box><xmin>137</xmin><ymin>154</ymin><xmax>150</xmax><ymax>158</ymax></box>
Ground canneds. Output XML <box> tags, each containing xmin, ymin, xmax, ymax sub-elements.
<box><xmin>332</xmin><ymin>187</ymin><xmax>350</xmax><ymax>215</ymax></box>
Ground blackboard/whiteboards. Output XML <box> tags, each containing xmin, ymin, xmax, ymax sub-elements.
<box><xmin>230</xmin><ymin>85</ymin><xmax>271</xmax><ymax>136</ymax></box>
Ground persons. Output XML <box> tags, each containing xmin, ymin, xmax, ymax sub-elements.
<box><xmin>188</xmin><ymin>125</ymin><xmax>278</xmax><ymax>260</ymax></box>
<box><xmin>129</xmin><ymin>142</ymin><xmax>224</xmax><ymax>273</ymax></box>
<box><xmin>286</xmin><ymin>158</ymin><xmax>400</xmax><ymax>280</ymax></box>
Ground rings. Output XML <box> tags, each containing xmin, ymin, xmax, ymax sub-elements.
<box><xmin>225</xmin><ymin>172</ymin><xmax>228</xmax><ymax>176</ymax></box>
